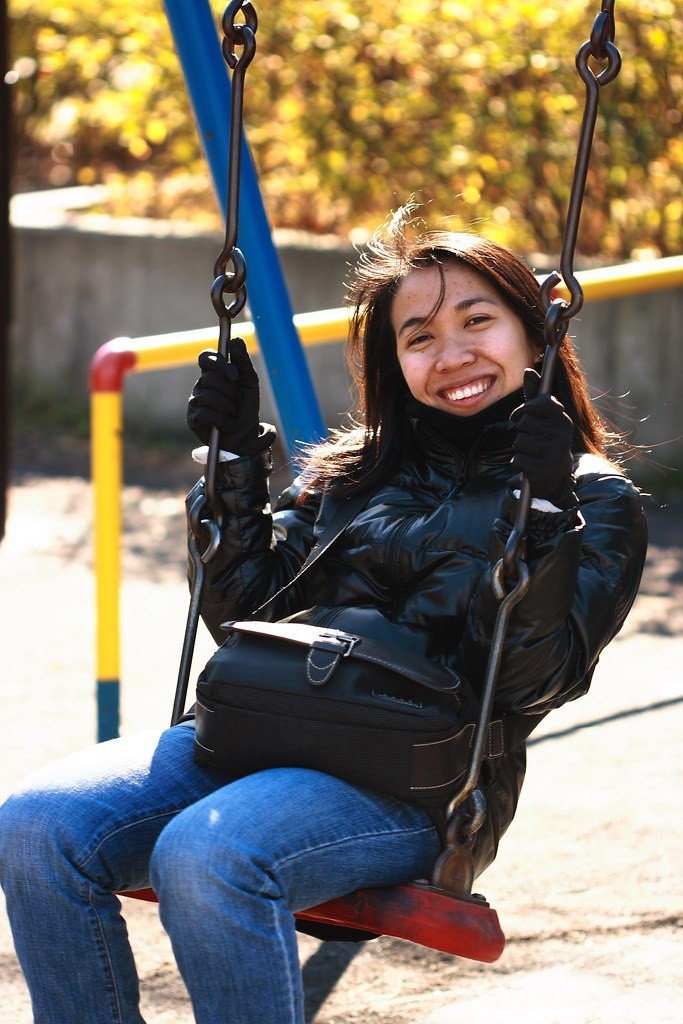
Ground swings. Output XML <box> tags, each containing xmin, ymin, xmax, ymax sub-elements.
<box><xmin>118</xmin><ymin>0</ymin><xmax>627</xmax><ymax>965</ymax></box>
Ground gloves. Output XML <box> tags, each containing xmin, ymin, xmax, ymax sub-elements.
<box><xmin>187</xmin><ymin>337</ymin><xmax>278</xmax><ymax>455</ymax></box>
<box><xmin>509</xmin><ymin>367</ymin><xmax>580</xmax><ymax>510</ymax></box>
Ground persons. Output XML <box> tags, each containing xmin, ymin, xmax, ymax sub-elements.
<box><xmin>0</xmin><ymin>232</ymin><xmax>648</xmax><ymax>1024</ymax></box>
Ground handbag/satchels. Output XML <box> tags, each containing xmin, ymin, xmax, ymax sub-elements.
<box><xmin>194</xmin><ymin>620</ymin><xmax>488</xmax><ymax>808</ymax></box>
